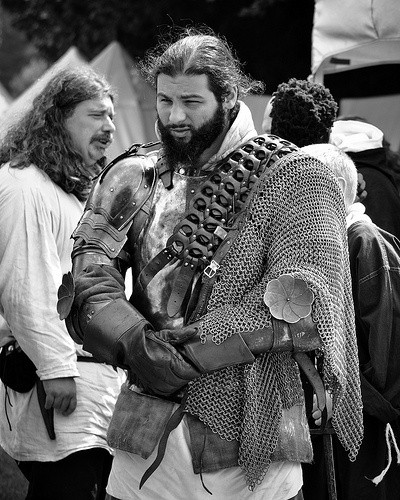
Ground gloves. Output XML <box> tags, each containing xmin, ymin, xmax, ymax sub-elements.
<box><xmin>128</xmin><ymin>307</ymin><xmax>255</xmax><ymax>394</ymax></box>
<box><xmin>81</xmin><ymin>297</ymin><xmax>200</xmax><ymax>396</ymax></box>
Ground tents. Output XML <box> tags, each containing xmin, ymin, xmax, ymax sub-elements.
<box><xmin>0</xmin><ymin>41</ymin><xmax>158</xmax><ymax>165</ymax></box>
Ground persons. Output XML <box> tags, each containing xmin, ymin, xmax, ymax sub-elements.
<box><xmin>0</xmin><ymin>71</ymin><xmax>132</xmax><ymax>500</ymax></box>
<box><xmin>57</xmin><ymin>25</ymin><xmax>363</xmax><ymax>500</ymax></box>
<box><xmin>261</xmin><ymin>77</ymin><xmax>400</xmax><ymax>500</ymax></box>
